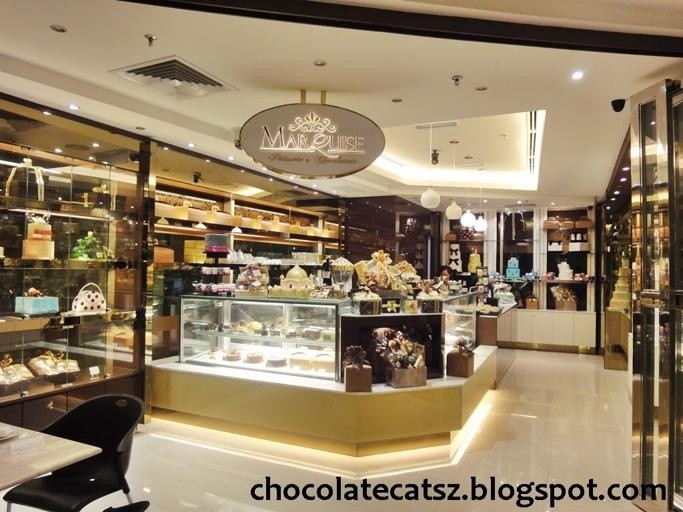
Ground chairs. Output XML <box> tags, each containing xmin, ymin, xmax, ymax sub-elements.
<box><xmin>3</xmin><ymin>393</ymin><xmax>145</xmax><ymax>512</ymax></box>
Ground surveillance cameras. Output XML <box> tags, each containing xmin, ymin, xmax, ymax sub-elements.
<box><xmin>611</xmin><ymin>99</ymin><xmax>626</xmax><ymax>112</ymax></box>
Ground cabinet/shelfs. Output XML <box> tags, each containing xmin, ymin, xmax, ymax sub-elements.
<box><xmin>490</xmin><ymin>201</ymin><xmax>595</xmax><ymax>314</ymax></box>
<box><xmin>0</xmin><ymin>93</ymin><xmax>151</xmax><ymax>432</ymax></box>
<box><xmin>0</xmin><ymin>373</ymin><xmax>144</xmax><ymax>433</ymax></box>
<box><xmin>496</xmin><ymin>309</ymin><xmax>598</xmax><ymax>356</ymax></box>
<box><xmin>345</xmin><ymin>195</ymin><xmax>440</xmax><ymax>280</ymax></box>
<box><xmin>416</xmin><ymin>292</ymin><xmax>477</xmax><ymax>372</ymax></box>
<box><xmin>155</xmin><ymin>176</ymin><xmax>340</xmax><ymax>271</ymax></box>
<box><xmin>177</xmin><ymin>295</ymin><xmax>350</xmax><ymax>383</ymax></box>
<box><xmin>597</xmin><ymin>124</ymin><xmax>632</xmax><ymax>372</ymax></box>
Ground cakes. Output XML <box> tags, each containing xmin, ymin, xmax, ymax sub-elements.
<box><xmin>288</xmin><ymin>352</ymin><xmax>311</xmax><ymax>370</ymax></box>
<box><xmin>506</xmin><ymin>256</ymin><xmax>520</xmax><ymax>279</ymax></box>
<box><xmin>266</xmin><ymin>351</ymin><xmax>287</xmax><ymax>368</ymax></box>
<box><xmin>414</xmin><ymin>240</ymin><xmax>427</xmax><ymax>269</ymax></box>
<box><xmin>22</xmin><ymin>217</ymin><xmax>54</xmax><ymax>260</ymax></box>
<box><xmin>449</xmin><ymin>244</ymin><xmax>462</xmax><ymax>273</ymax></box>
<box><xmin>311</xmin><ymin>353</ymin><xmax>335</xmax><ymax>373</ymax></box>
<box><xmin>209</xmin><ymin>344</ymin><xmax>221</xmax><ymax>361</ymax></box>
<box><xmin>468</xmin><ymin>249</ymin><xmax>481</xmax><ymax>273</ymax></box>
<box><xmin>227</xmin><ymin>349</ymin><xmax>241</xmax><ymax>361</ymax></box>
<box><xmin>247</xmin><ymin>352</ymin><xmax>263</xmax><ymax>364</ymax></box>
<box><xmin>14</xmin><ymin>286</ymin><xmax>60</xmax><ymax>314</ymax></box>
<box><xmin>610</xmin><ymin>251</ymin><xmax>631</xmax><ymax>309</ymax></box>
<box><xmin>207</xmin><ymin>320</ymin><xmax>335</xmax><ymax>341</ymax></box>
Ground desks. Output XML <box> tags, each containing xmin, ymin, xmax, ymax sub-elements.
<box><xmin>0</xmin><ymin>421</ymin><xmax>102</xmax><ymax>490</ymax></box>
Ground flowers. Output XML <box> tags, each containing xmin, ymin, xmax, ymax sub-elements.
<box><xmin>371</xmin><ymin>326</ymin><xmax>426</xmax><ymax>369</ymax></box>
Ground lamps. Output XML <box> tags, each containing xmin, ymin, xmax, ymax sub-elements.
<box><xmin>421</xmin><ymin>124</ymin><xmax>488</xmax><ymax>232</ymax></box>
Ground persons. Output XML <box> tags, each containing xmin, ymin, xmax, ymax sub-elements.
<box><xmin>439</xmin><ymin>265</ymin><xmax>454</xmax><ymax>280</ymax></box>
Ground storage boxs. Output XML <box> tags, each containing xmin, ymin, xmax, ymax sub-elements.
<box><xmin>446</xmin><ymin>337</ymin><xmax>474</xmax><ymax>378</ymax></box>
<box><xmin>341</xmin><ymin>345</ymin><xmax>372</xmax><ymax>392</ymax></box>
<box><xmin>556</xmin><ymin>300</ymin><xmax>577</xmax><ymax>310</ymax></box>
<box><xmin>384</xmin><ymin>365</ymin><xmax>428</xmax><ymax>388</ymax></box>
